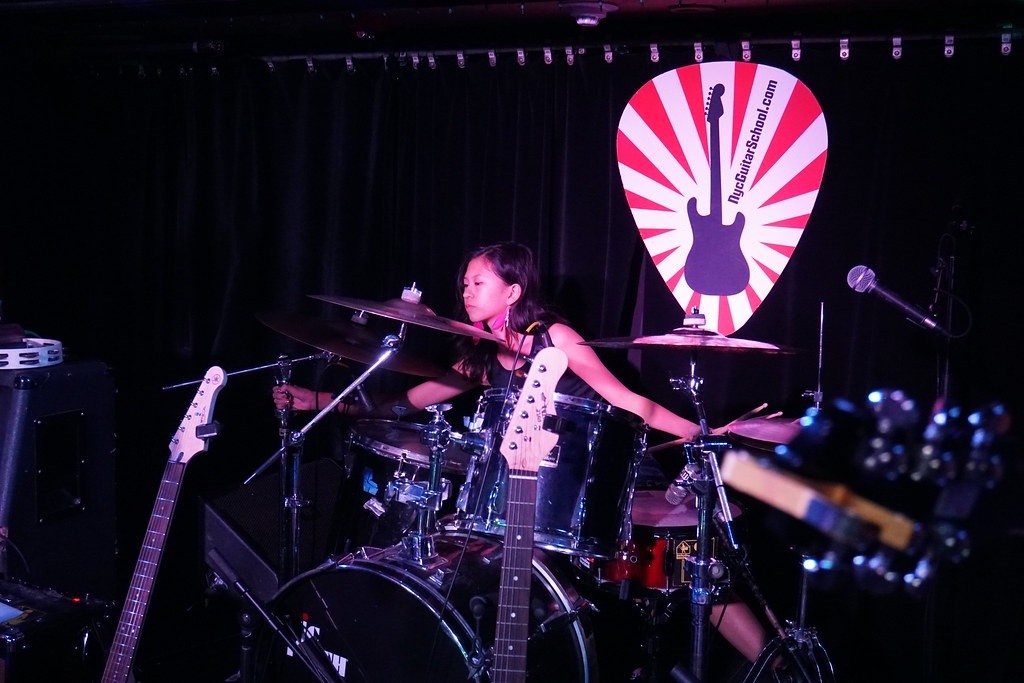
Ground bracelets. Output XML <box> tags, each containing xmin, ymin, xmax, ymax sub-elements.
<box><xmin>316</xmin><ymin>390</ymin><xmax>320</xmax><ymax>412</ymax></box>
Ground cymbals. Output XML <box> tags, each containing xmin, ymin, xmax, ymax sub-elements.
<box><xmin>575</xmin><ymin>327</ymin><xmax>803</xmax><ymax>357</ymax></box>
<box><xmin>0</xmin><ymin>338</ymin><xmax>64</xmax><ymax>370</ymax></box>
<box><xmin>726</xmin><ymin>418</ymin><xmax>804</xmax><ymax>454</ymax></box>
<box><xmin>306</xmin><ymin>292</ymin><xmax>507</xmax><ymax>344</ymax></box>
<box><xmin>256</xmin><ymin>312</ymin><xmax>449</xmax><ymax>379</ymax></box>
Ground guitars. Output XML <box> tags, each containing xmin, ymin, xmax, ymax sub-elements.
<box><xmin>100</xmin><ymin>365</ymin><xmax>229</xmax><ymax>683</ymax></box>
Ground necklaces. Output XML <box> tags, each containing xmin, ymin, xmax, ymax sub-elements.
<box><xmin>490</xmin><ymin>327</ymin><xmax>506</xmax><ymax>335</ymax></box>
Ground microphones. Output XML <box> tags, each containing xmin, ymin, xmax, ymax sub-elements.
<box><xmin>847</xmin><ymin>266</ymin><xmax>942</xmax><ymax>331</ymax></box>
<box><xmin>666</xmin><ymin>460</ymin><xmax>699</xmax><ymax>506</ymax></box>
<box><xmin>536</xmin><ymin>321</ymin><xmax>554</xmax><ymax>349</ymax></box>
<box><xmin>352</xmin><ymin>375</ymin><xmax>377</xmax><ymax>417</ymax></box>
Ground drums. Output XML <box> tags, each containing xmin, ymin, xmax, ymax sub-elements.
<box><xmin>449</xmin><ymin>387</ymin><xmax>649</xmax><ymax>562</ymax></box>
<box><xmin>602</xmin><ymin>484</ymin><xmax>751</xmax><ymax>596</ymax></box>
<box><xmin>250</xmin><ymin>531</ymin><xmax>590</xmax><ymax>683</ymax></box>
<box><xmin>321</xmin><ymin>417</ymin><xmax>471</xmax><ymax>554</ymax></box>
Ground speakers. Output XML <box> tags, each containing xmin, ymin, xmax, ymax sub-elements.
<box><xmin>0</xmin><ymin>338</ymin><xmax>124</xmax><ymax>606</ymax></box>
<box><xmin>199</xmin><ymin>457</ymin><xmax>380</xmax><ymax>604</ymax></box>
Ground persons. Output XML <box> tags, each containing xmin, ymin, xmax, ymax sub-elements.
<box><xmin>273</xmin><ymin>241</ymin><xmax>782</xmax><ymax>673</ymax></box>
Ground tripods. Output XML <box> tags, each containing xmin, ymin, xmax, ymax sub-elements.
<box><xmin>744</xmin><ymin>549</ymin><xmax>837</xmax><ymax>683</ymax></box>
